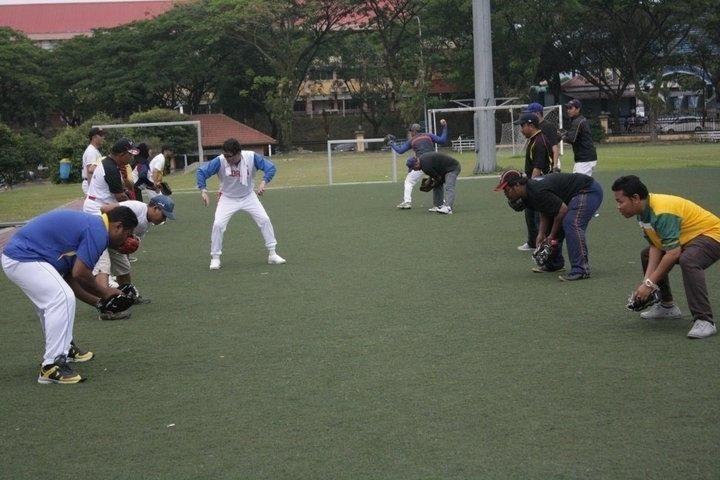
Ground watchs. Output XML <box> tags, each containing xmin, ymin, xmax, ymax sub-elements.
<box><xmin>645</xmin><ymin>278</ymin><xmax>660</xmax><ymax>292</ymax></box>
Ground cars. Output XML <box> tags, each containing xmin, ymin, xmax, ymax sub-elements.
<box><xmin>660</xmin><ymin>116</ymin><xmax>703</xmax><ymax>134</ymax></box>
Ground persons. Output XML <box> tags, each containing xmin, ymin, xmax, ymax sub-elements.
<box><xmin>406</xmin><ymin>151</ymin><xmax>461</xmax><ymax>215</ymax></box>
<box><xmin>81</xmin><ymin>126</ymin><xmax>176</xmax><ymax>321</ymax></box>
<box><xmin>521</xmin><ymin>102</ymin><xmax>561</xmax><ymax>173</ymax></box>
<box><xmin>508</xmin><ymin>114</ymin><xmax>554</xmax><ymax>251</ymax></box>
<box><xmin>556</xmin><ymin>98</ymin><xmax>600</xmax><ymax>218</ymax></box>
<box><xmin>612</xmin><ymin>175</ymin><xmax>720</xmax><ymax>338</ymax></box>
<box><xmin>1</xmin><ymin>205</ymin><xmax>138</xmax><ymax>384</ymax></box>
<box><xmin>197</xmin><ymin>138</ymin><xmax>286</xmax><ymax>270</ymax></box>
<box><xmin>493</xmin><ymin>169</ymin><xmax>604</xmax><ymax>281</ymax></box>
<box><xmin>385</xmin><ymin>119</ymin><xmax>448</xmax><ymax>209</ymax></box>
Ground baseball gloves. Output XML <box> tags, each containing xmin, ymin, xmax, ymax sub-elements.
<box><xmin>102</xmin><ymin>281</ymin><xmax>140</xmax><ymax>311</ymax></box>
<box><xmin>507</xmin><ymin>196</ymin><xmax>525</xmax><ymax>211</ymax></box>
<box><xmin>558</xmin><ymin>129</ymin><xmax>568</xmax><ymax>139</ymax></box>
<box><xmin>116</xmin><ymin>232</ymin><xmax>141</xmax><ymax>254</ymax></box>
<box><xmin>625</xmin><ymin>282</ymin><xmax>663</xmax><ymax>311</ymax></box>
<box><xmin>419</xmin><ymin>179</ymin><xmax>434</xmax><ymax>191</ymax></box>
<box><xmin>533</xmin><ymin>237</ymin><xmax>558</xmax><ymax>268</ymax></box>
<box><xmin>384</xmin><ymin>133</ymin><xmax>396</xmax><ymax>148</ymax></box>
<box><xmin>158</xmin><ymin>181</ymin><xmax>173</xmax><ymax>196</ymax></box>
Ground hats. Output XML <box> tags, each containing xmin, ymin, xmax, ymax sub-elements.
<box><xmin>522</xmin><ymin>103</ymin><xmax>544</xmax><ymax>114</ymax></box>
<box><xmin>409</xmin><ymin>124</ymin><xmax>421</xmax><ymax>131</ymax></box>
<box><xmin>88</xmin><ymin>128</ymin><xmax>106</xmax><ymax>139</ymax></box>
<box><xmin>113</xmin><ymin>137</ymin><xmax>140</xmax><ymax>155</ymax></box>
<box><xmin>512</xmin><ymin>113</ymin><xmax>538</xmax><ymax>125</ymax></box>
<box><xmin>406</xmin><ymin>156</ymin><xmax>416</xmax><ymax>173</ymax></box>
<box><xmin>152</xmin><ymin>195</ymin><xmax>176</xmax><ymax>220</ymax></box>
<box><xmin>565</xmin><ymin>99</ymin><xmax>582</xmax><ymax>108</ymax></box>
<box><xmin>494</xmin><ymin>169</ymin><xmax>522</xmax><ymax>191</ymax></box>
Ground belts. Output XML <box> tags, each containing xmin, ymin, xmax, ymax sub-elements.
<box><xmin>89</xmin><ymin>195</ymin><xmax>97</xmax><ymax>201</ymax></box>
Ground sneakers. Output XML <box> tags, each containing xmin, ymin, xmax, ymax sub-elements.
<box><xmin>429</xmin><ymin>206</ymin><xmax>453</xmax><ymax>215</ymax></box>
<box><xmin>640</xmin><ymin>303</ymin><xmax>682</xmax><ymax>319</ymax></box>
<box><xmin>268</xmin><ymin>254</ymin><xmax>286</xmax><ymax>265</ymax></box>
<box><xmin>66</xmin><ymin>340</ymin><xmax>96</xmax><ymax>363</ymax></box>
<box><xmin>100</xmin><ymin>310</ymin><xmax>130</xmax><ymax>320</ymax></box>
<box><xmin>532</xmin><ymin>266</ymin><xmax>567</xmax><ymax>273</ymax></box>
<box><xmin>397</xmin><ymin>202</ymin><xmax>413</xmax><ymax>209</ymax></box>
<box><xmin>37</xmin><ymin>356</ymin><xmax>82</xmax><ymax>384</ymax></box>
<box><xmin>210</xmin><ymin>259</ymin><xmax>221</xmax><ymax>270</ymax></box>
<box><xmin>687</xmin><ymin>319</ymin><xmax>717</xmax><ymax>338</ymax></box>
<box><xmin>135</xmin><ymin>295</ymin><xmax>152</xmax><ymax>305</ymax></box>
<box><xmin>559</xmin><ymin>273</ymin><xmax>591</xmax><ymax>281</ymax></box>
<box><xmin>518</xmin><ymin>243</ymin><xmax>535</xmax><ymax>251</ymax></box>
